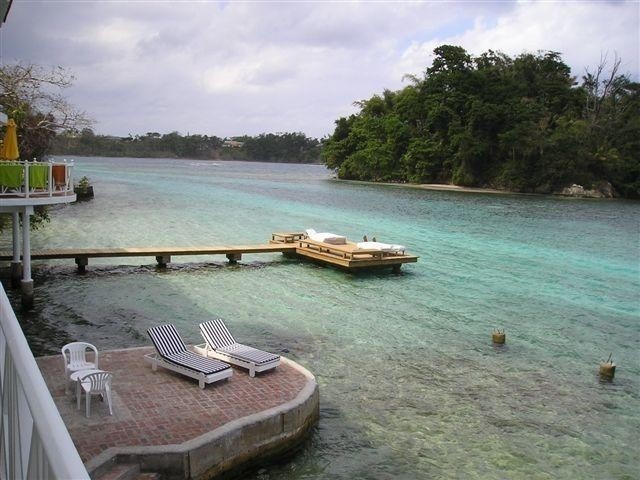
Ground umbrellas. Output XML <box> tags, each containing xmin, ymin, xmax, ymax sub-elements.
<box><xmin>0</xmin><ymin>117</ymin><xmax>20</xmax><ymax>161</ymax></box>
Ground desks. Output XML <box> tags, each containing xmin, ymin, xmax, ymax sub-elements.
<box><xmin>69</xmin><ymin>370</ymin><xmax>108</xmax><ymax>398</ymax></box>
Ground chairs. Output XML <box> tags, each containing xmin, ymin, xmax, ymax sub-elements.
<box><xmin>143</xmin><ymin>323</ymin><xmax>233</xmax><ymax>389</ymax></box>
<box><xmin>61</xmin><ymin>341</ymin><xmax>99</xmax><ymax>394</ymax></box>
<box><xmin>194</xmin><ymin>318</ymin><xmax>280</xmax><ymax>378</ymax></box>
<box><xmin>76</xmin><ymin>371</ymin><xmax>113</xmax><ymax>418</ymax></box>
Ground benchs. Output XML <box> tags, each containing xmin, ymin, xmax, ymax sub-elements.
<box><xmin>285</xmin><ymin>232</ymin><xmax>304</xmax><ymax>239</ymax></box>
<box><xmin>272</xmin><ymin>233</ymin><xmax>294</xmax><ymax>243</ymax></box>
<box><xmin>306</xmin><ymin>228</ymin><xmax>346</xmax><ymax>245</ymax></box>
<box><xmin>356</xmin><ymin>241</ymin><xmax>405</xmax><ymax>256</ymax></box>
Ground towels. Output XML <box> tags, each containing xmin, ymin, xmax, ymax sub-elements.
<box><xmin>29</xmin><ymin>164</ymin><xmax>49</xmax><ymax>188</ymax></box>
<box><xmin>0</xmin><ymin>163</ymin><xmax>25</xmax><ymax>188</ymax></box>
<box><xmin>53</xmin><ymin>165</ymin><xmax>66</xmax><ymax>187</ymax></box>
